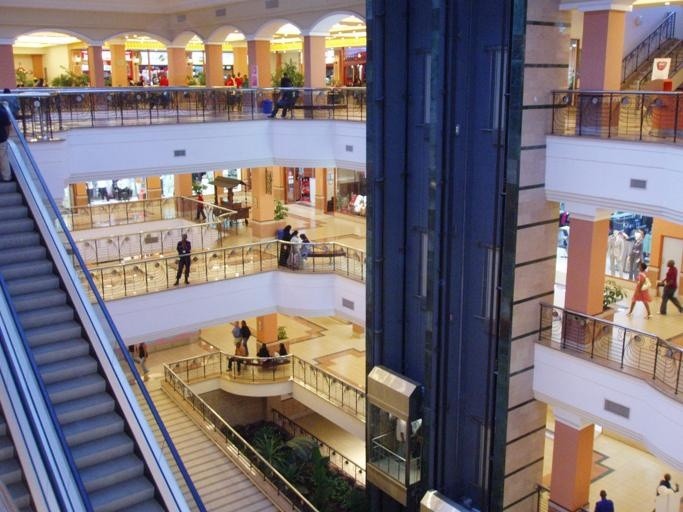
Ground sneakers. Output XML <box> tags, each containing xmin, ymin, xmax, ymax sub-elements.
<box><xmin>227</xmin><ymin>368</ymin><xmax>232</xmax><ymax>372</ymax></box>
<box><xmin>196</xmin><ymin>217</ymin><xmax>206</xmax><ymax>220</ymax></box>
<box><xmin>174</xmin><ymin>278</ymin><xmax>189</xmax><ymax>285</ymax></box>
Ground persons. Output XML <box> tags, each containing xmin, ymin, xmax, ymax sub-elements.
<box><xmin>138</xmin><ymin>342</ymin><xmax>150</xmax><ymax>374</ymax></box>
<box><xmin>268</xmin><ymin>81</ymin><xmax>298</xmax><ymax>118</ymax></box>
<box><xmin>97</xmin><ymin>180</ymin><xmax>110</xmax><ymax>201</ymax></box>
<box><xmin>352</xmin><ymin>74</ymin><xmax>362</xmax><ymax>99</ymax></box>
<box><xmin>594</xmin><ymin>490</ymin><xmax>614</xmax><ymax>512</ymax></box>
<box><xmin>226</xmin><ymin>320</ymin><xmax>288</xmax><ymax>376</ymax></box>
<box><xmin>277</xmin><ymin>225</ymin><xmax>310</xmax><ymax>270</ymax></box>
<box><xmin>652</xmin><ymin>474</ymin><xmax>679</xmax><ymax>512</ymax></box>
<box><xmin>129</xmin><ymin>345</ymin><xmax>140</xmax><ymax>364</ymax></box>
<box><xmin>657</xmin><ymin>260</ymin><xmax>683</xmax><ymax>315</ymax></box>
<box><xmin>396</xmin><ymin>417</ymin><xmax>406</xmax><ymax>458</ymax></box>
<box><xmin>127</xmin><ymin>71</ymin><xmax>169</xmax><ymax>87</ymax></box>
<box><xmin>195</xmin><ymin>192</ymin><xmax>207</xmax><ymax>220</ymax></box>
<box><xmin>0</xmin><ymin>77</ymin><xmax>50</xmax><ymax>118</ymax></box>
<box><xmin>624</xmin><ymin>263</ymin><xmax>653</xmax><ymax>320</ymax></box>
<box><xmin>326</xmin><ymin>75</ymin><xmax>331</xmax><ymax>85</ymax></box>
<box><xmin>281</xmin><ymin>72</ymin><xmax>292</xmax><ymax>87</ymax></box>
<box><xmin>225</xmin><ymin>72</ymin><xmax>249</xmax><ymax>89</ymax></box>
<box><xmin>0</xmin><ymin>102</ymin><xmax>14</xmax><ymax>182</ymax></box>
<box><xmin>173</xmin><ymin>233</ymin><xmax>191</xmax><ymax>285</ymax></box>
<box><xmin>639</xmin><ymin>228</ymin><xmax>650</xmax><ymax>261</ymax></box>
<box><xmin>608</xmin><ymin>229</ymin><xmax>643</xmax><ymax>280</ymax></box>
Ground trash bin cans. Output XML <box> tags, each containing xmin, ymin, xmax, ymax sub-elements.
<box><xmin>264</xmin><ymin>101</ymin><xmax>273</xmax><ymax>112</ymax></box>
<box><xmin>278</xmin><ymin>231</ymin><xmax>286</xmax><ymax>239</ymax></box>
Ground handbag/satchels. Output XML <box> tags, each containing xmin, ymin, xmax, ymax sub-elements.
<box><xmin>638</xmin><ymin>277</ymin><xmax>652</xmax><ymax>291</ymax></box>
<box><xmin>657</xmin><ymin>281</ymin><xmax>665</xmax><ymax>287</ymax></box>
<box><xmin>233</xmin><ymin>337</ymin><xmax>242</xmax><ymax>344</ymax></box>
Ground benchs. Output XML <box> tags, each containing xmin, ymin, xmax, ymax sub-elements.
<box><xmin>304</xmin><ymin>249</ymin><xmax>346</xmax><ymax>265</ymax></box>
<box><xmin>226</xmin><ymin>353</ymin><xmax>291</xmax><ymax>381</ymax></box>
<box><xmin>279</xmin><ymin>101</ymin><xmax>350</xmax><ymax>121</ymax></box>
